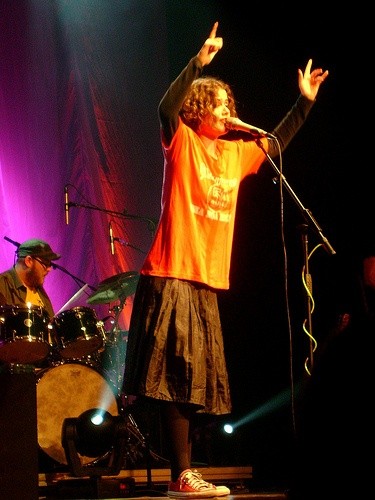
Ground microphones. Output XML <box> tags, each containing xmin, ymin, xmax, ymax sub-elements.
<box><xmin>109</xmin><ymin>224</ymin><xmax>115</xmax><ymax>255</ymax></box>
<box><xmin>226</xmin><ymin>117</ymin><xmax>277</xmax><ymax>140</ymax></box>
<box><xmin>64</xmin><ymin>187</ymin><xmax>69</xmax><ymax>225</ymax></box>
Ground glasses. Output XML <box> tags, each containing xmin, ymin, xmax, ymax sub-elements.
<box><xmin>32</xmin><ymin>257</ymin><xmax>59</xmax><ymax>270</ymax></box>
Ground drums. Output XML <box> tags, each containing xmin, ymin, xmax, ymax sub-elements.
<box><xmin>0</xmin><ymin>303</ymin><xmax>48</xmax><ymax>364</ymax></box>
<box><xmin>51</xmin><ymin>305</ymin><xmax>104</xmax><ymax>360</ymax></box>
<box><xmin>37</xmin><ymin>359</ymin><xmax>122</xmax><ymax>468</ymax></box>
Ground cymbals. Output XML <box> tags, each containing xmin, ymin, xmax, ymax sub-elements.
<box><xmin>86</xmin><ymin>271</ymin><xmax>141</xmax><ymax>304</ymax></box>
<box><xmin>105</xmin><ymin>328</ymin><xmax>133</xmax><ymax>338</ymax></box>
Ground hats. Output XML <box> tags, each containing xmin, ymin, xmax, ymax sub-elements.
<box><xmin>15</xmin><ymin>239</ymin><xmax>61</xmax><ymax>261</ymax></box>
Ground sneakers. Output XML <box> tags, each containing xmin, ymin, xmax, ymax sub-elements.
<box><xmin>167</xmin><ymin>469</ymin><xmax>231</xmax><ymax>498</ymax></box>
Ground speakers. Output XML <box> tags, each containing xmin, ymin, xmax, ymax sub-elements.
<box><xmin>0</xmin><ymin>372</ymin><xmax>40</xmax><ymax>500</ymax></box>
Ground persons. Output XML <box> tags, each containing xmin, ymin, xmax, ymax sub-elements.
<box><xmin>0</xmin><ymin>240</ymin><xmax>61</xmax><ymax>319</ymax></box>
<box><xmin>320</xmin><ymin>255</ymin><xmax>375</xmax><ymax>342</ymax></box>
<box><xmin>123</xmin><ymin>20</ymin><xmax>329</xmax><ymax>499</ymax></box>
